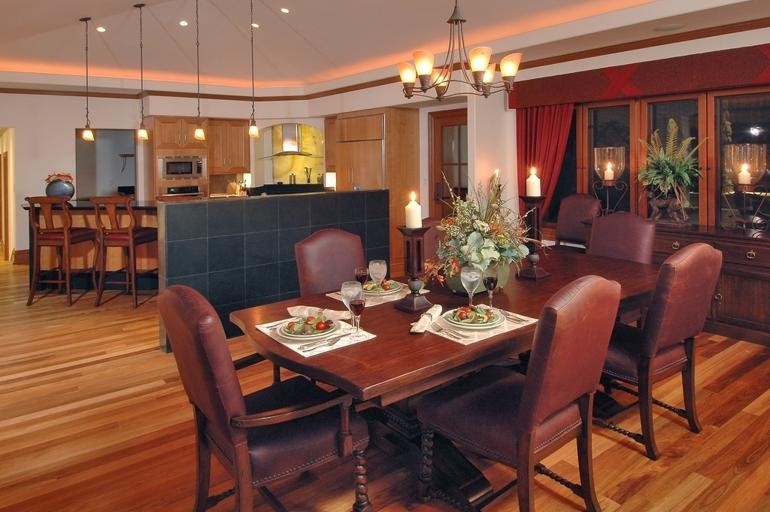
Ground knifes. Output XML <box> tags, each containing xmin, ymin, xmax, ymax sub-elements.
<box><xmin>299</xmin><ymin>333</ymin><xmax>352</xmax><ymax>350</ymax></box>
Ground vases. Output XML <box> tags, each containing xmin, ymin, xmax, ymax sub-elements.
<box><xmin>46</xmin><ymin>182</ymin><xmax>74</xmax><ymax>208</ymax></box>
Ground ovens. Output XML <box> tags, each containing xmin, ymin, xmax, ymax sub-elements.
<box><xmin>158</xmin><ymin>185</ymin><xmax>204</xmax><ymax>200</ymax></box>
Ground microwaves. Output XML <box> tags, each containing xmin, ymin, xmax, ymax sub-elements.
<box><xmin>159</xmin><ymin>157</ymin><xmax>203</xmax><ymax>179</ymax></box>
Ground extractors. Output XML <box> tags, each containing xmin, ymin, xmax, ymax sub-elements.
<box><xmin>259</xmin><ymin>124</ymin><xmax>324</xmax><ymax>160</ymax></box>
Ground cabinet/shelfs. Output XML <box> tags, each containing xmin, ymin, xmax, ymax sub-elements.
<box><xmin>334</xmin><ymin>107</ymin><xmax>420</xmax><ymax>278</ymax></box>
<box><xmin>144</xmin><ymin>115</ymin><xmax>206</xmax><ymax>150</ymax></box>
<box><xmin>656</xmin><ymin>232</ymin><xmax>770</xmax><ymax>346</ymax></box>
<box><xmin>324</xmin><ymin>116</ymin><xmax>337</xmax><ymax>172</ymax></box>
<box><xmin>207</xmin><ymin>118</ymin><xmax>250</xmax><ymax>176</ymax></box>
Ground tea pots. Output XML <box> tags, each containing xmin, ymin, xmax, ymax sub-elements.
<box><xmin>289</xmin><ymin>173</ymin><xmax>296</xmax><ymax>184</ymax></box>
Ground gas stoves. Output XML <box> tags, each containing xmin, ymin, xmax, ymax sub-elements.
<box><xmin>251</xmin><ymin>182</ymin><xmax>324</xmax><ymax>195</ymax></box>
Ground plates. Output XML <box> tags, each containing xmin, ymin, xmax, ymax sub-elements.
<box><xmin>441</xmin><ymin>306</ymin><xmax>507</xmax><ymax>329</ymax></box>
<box><xmin>276</xmin><ymin>318</ymin><xmax>341</xmax><ymax>339</ymax></box>
<box><xmin>364</xmin><ymin>281</ymin><xmax>400</xmax><ymax>293</ymax></box>
<box><xmin>280</xmin><ymin>320</ymin><xmax>336</xmax><ymax>337</ymax></box>
<box><xmin>364</xmin><ymin>281</ymin><xmax>403</xmax><ymax>296</ymax></box>
<box><xmin>447</xmin><ymin>313</ymin><xmax>501</xmax><ymax>327</ymax></box>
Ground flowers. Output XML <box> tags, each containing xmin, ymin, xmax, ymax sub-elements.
<box><xmin>420</xmin><ymin>168</ymin><xmax>552</xmax><ymax>287</ymax></box>
<box><xmin>45</xmin><ymin>172</ymin><xmax>73</xmax><ymax>184</ymax></box>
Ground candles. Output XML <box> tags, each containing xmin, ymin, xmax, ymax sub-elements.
<box><xmin>739</xmin><ymin>163</ymin><xmax>752</xmax><ymax>184</ymax></box>
<box><xmin>525</xmin><ymin>167</ymin><xmax>541</xmax><ymax>196</ymax></box>
<box><xmin>604</xmin><ymin>161</ymin><xmax>614</xmax><ymax>180</ymax></box>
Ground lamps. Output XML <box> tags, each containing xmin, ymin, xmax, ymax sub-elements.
<box><xmin>78</xmin><ymin>0</ymin><xmax>259</xmax><ymax>143</ymax></box>
<box><xmin>397</xmin><ymin>0</ymin><xmax>523</xmax><ymax>103</ymax></box>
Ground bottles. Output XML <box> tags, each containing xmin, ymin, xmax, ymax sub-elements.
<box><xmin>316</xmin><ymin>173</ymin><xmax>324</xmax><ymax>185</ymax></box>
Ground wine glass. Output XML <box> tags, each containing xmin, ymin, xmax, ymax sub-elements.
<box><xmin>353</xmin><ymin>263</ymin><xmax>368</xmax><ymax>287</ymax></box>
<box><xmin>348</xmin><ymin>288</ymin><xmax>366</xmax><ymax>341</ymax></box>
<box><xmin>483</xmin><ymin>270</ymin><xmax>498</xmax><ymax>308</ymax></box>
<box><xmin>460</xmin><ymin>267</ymin><xmax>482</xmax><ymax>305</ymax></box>
<box><xmin>340</xmin><ymin>280</ymin><xmax>363</xmax><ymax>334</ymax></box>
<box><xmin>368</xmin><ymin>260</ymin><xmax>387</xmax><ymax>296</ymax></box>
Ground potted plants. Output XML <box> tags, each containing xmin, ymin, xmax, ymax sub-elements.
<box><xmin>633</xmin><ymin>119</ymin><xmax>710</xmax><ymax>224</ymax></box>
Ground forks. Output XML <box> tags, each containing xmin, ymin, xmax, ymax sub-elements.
<box><xmin>430</xmin><ymin>321</ymin><xmax>461</xmax><ymax>340</ymax></box>
<box><xmin>303</xmin><ymin>336</ymin><xmax>340</xmax><ymax>354</ymax></box>
